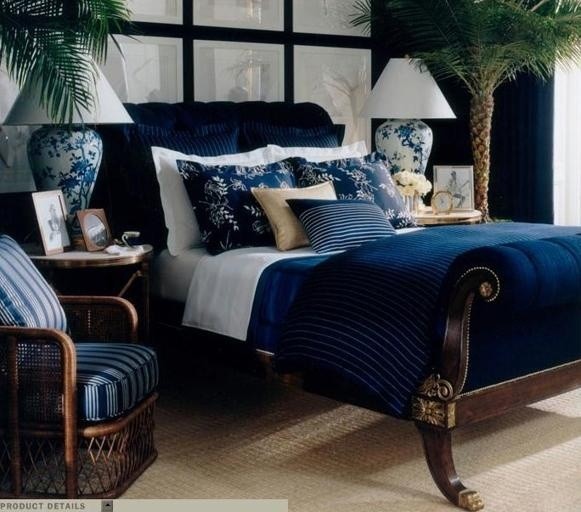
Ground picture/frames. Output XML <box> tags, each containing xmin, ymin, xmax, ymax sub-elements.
<box><xmin>432</xmin><ymin>164</ymin><xmax>476</xmax><ymax>214</ymax></box>
<box><xmin>30</xmin><ymin>188</ymin><xmax>115</xmax><ymax>257</ymax></box>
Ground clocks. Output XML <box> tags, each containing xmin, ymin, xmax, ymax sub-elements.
<box><xmin>431</xmin><ymin>190</ymin><xmax>454</xmax><ymax>216</ymax></box>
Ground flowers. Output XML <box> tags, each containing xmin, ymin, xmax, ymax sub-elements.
<box><xmin>390</xmin><ymin>170</ymin><xmax>433</xmax><ymax>200</ymax></box>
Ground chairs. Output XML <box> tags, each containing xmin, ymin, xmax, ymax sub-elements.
<box><xmin>0</xmin><ymin>233</ymin><xmax>161</xmax><ymax>499</ymax></box>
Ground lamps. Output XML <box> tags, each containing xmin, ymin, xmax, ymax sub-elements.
<box><xmin>0</xmin><ymin>41</ymin><xmax>136</xmax><ymax>241</ymax></box>
<box><xmin>356</xmin><ymin>55</ymin><xmax>461</xmax><ymax>175</ymax></box>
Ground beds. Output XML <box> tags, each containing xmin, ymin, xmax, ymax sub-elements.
<box><xmin>87</xmin><ymin>101</ymin><xmax>581</xmax><ymax>511</ymax></box>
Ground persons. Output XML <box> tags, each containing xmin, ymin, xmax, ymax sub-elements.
<box><xmin>446</xmin><ymin>171</ymin><xmax>466</xmax><ymax>208</ymax></box>
<box><xmin>45</xmin><ymin>202</ymin><xmax>68</xmax><ymax>245</ymax></box>
